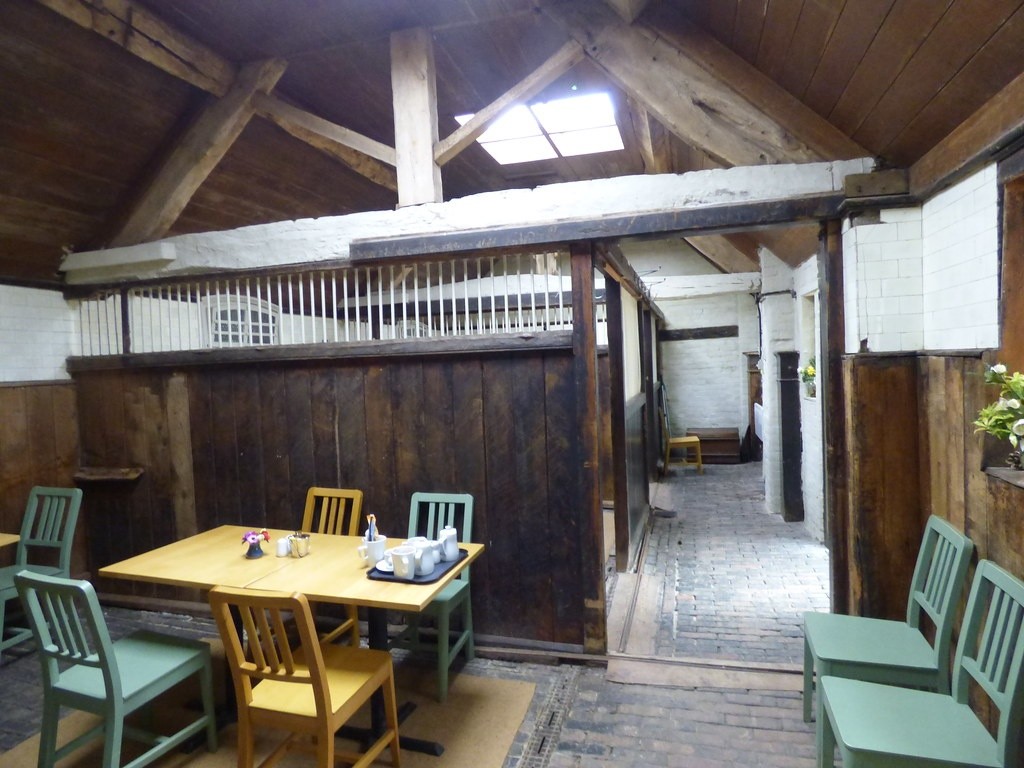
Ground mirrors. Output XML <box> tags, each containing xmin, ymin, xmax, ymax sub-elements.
<box><xmin>806</xmin><ymin>381</ymin><xmax>816</xmax><ymax>398</ymax></box>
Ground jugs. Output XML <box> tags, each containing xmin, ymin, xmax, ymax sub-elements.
<box><xmin>438</xmin><ymin>526</ymin><xmax>459</xmax><ymax>561</ymax></box>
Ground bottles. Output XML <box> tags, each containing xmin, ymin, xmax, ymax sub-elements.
<box><xmin>276</xmin><ymin>537</ymin><xmax>288</xmax><ymax>557</ymax></box>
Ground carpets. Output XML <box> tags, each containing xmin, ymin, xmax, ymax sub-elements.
<box><xmin>0</xmin><ymin>637</ymin><xmax>536</xmax><ymax>768</ymax></box>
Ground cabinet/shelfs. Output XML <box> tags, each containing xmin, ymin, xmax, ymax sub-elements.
<box><xmin>686</xmin><ymin>427</ymin><xmax>742</xmax><ymax>464</ymax></box>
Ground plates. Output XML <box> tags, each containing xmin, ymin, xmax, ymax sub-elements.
<box><xmin>375</xmin><ymin>560</ymin><xmax>394</xmax><ymax>572</ymax></box>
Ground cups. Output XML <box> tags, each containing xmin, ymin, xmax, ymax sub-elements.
<box><xmin>289</xmin><ymin>534</ymin><xmax>311</xmax><ymax>557</ymax></box>
<box><xmin>357</xmin><ymin>534</ymin><xmax>387</xmax><ymax>568</ymax></box>
<box><xmin>384</xmin><ymin>536</ymin><xmax>441</xmax><ymax>580</ymax></box>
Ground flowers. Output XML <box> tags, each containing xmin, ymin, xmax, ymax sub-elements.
<box><xmin>797</xmin><ymin>357</ymin><xmax>816</xmax><ymax>383</ymax></box>
<box><xmin>973</xmin><ymin>361</ymin><xmax>1024</xmax><ymax>452</ymax></box>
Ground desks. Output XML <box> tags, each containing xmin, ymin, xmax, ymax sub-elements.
<box><xmin>97</xmin><ymin>524</ymin><xmax>486</xmax><ymax>756</ymax></box>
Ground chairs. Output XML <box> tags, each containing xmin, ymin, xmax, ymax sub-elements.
<box><xmin>248</xmin><ymin>487</ymin><xmax>364</xmax><ymax>663</ymax></box>
<box><xmin>380</xmin><ymin>492</ymin><xmax>474</xmax><ymax>706</ymax></box>
<box><xmin>818</xmin><ymin>559</ymin><xmax>1024</xmax><ymax>768</ymax></box>
<box><xmin>13</xmin><ymin>570</ymin><xmax>218</xmax><ymax>768</ymax></box>
<box><xmin>658</xmin><ymin>406</ymin><xmax>703</xmax><ymax>477</ymax></box>
<box><xmin>209</xmin><ymin>585</ymin><xmax>402</xmax><ymax>768</ymax></box>
<box><xmin>0</xmin><ymin>485</ymin><xmax>84</xmax><ymax>653</ymax></box>
<box><xmin>801</xmin><ymin>514</ymin><xmax>974</xmax><ymax>721</ymax></box>
